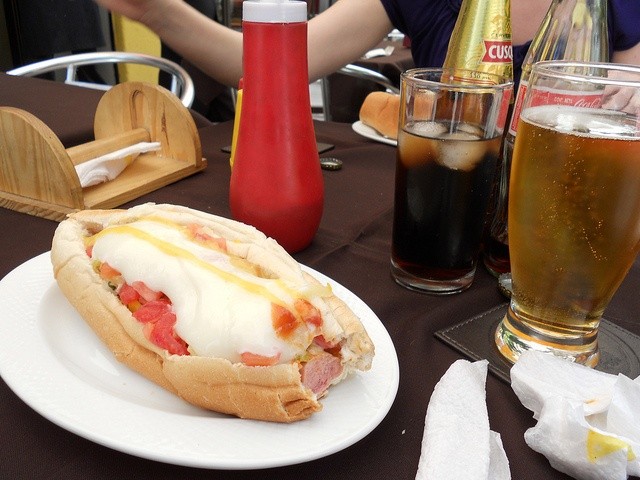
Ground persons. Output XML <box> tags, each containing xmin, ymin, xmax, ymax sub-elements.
<box><xmin>98</xmin><ymin>0</ymin><xmax>639</xmax><ymax>119</ymax></box>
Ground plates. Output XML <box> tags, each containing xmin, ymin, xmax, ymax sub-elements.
<box><xmin>0</xmin><ymin>249</ymin><xmax>400</xmax><ymax>472</ymax></box>
<box><xmin>351</xmin><ymin>120</ymin><xmax>398</xmax><ymax>146</ymax></box>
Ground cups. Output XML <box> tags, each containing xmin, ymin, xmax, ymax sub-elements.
<box><xmin>389</xmin><ymin>66</ymin><xmax>516</xmax><ymax>299</ymax></box>
<box><xmin>494</xmin><ymin>59</ymin><xmax>640</xmax><ymax>371</ymax></box>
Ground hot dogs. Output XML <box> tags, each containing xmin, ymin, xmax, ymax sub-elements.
<box><xmin>360</xmin><ymin>91</ymin><xmax>433</xmax><ymax>141</ymax></box>
<box><xmin>49</xmin><ymin>201</ymin><xmax>375</xmax><ymax>424</ymax></box>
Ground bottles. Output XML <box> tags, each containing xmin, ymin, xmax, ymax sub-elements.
<box><xmin>439</xmin><ymin>0</ymin><xmax>516</xmax><ymax>252</ymax></box>
<box><xmin>228</xmin><ymin>0</ymin><xmax>325</xmax><ymax>255</ymax></box>
<box><xmin>485</xmin><ymin>0</ymin><xmax>610</xmax><ymax>281</ymax></box>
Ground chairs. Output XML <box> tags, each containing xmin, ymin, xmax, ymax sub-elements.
<box><xmin>319</xmin><ymin>63</ymin><xmax>406</xmax><ymax>124</ymax></box>
<box><xmin>6</xmin><ymin>51</ymin><xmax>196</xmax><ymax>111</ymax></box>
